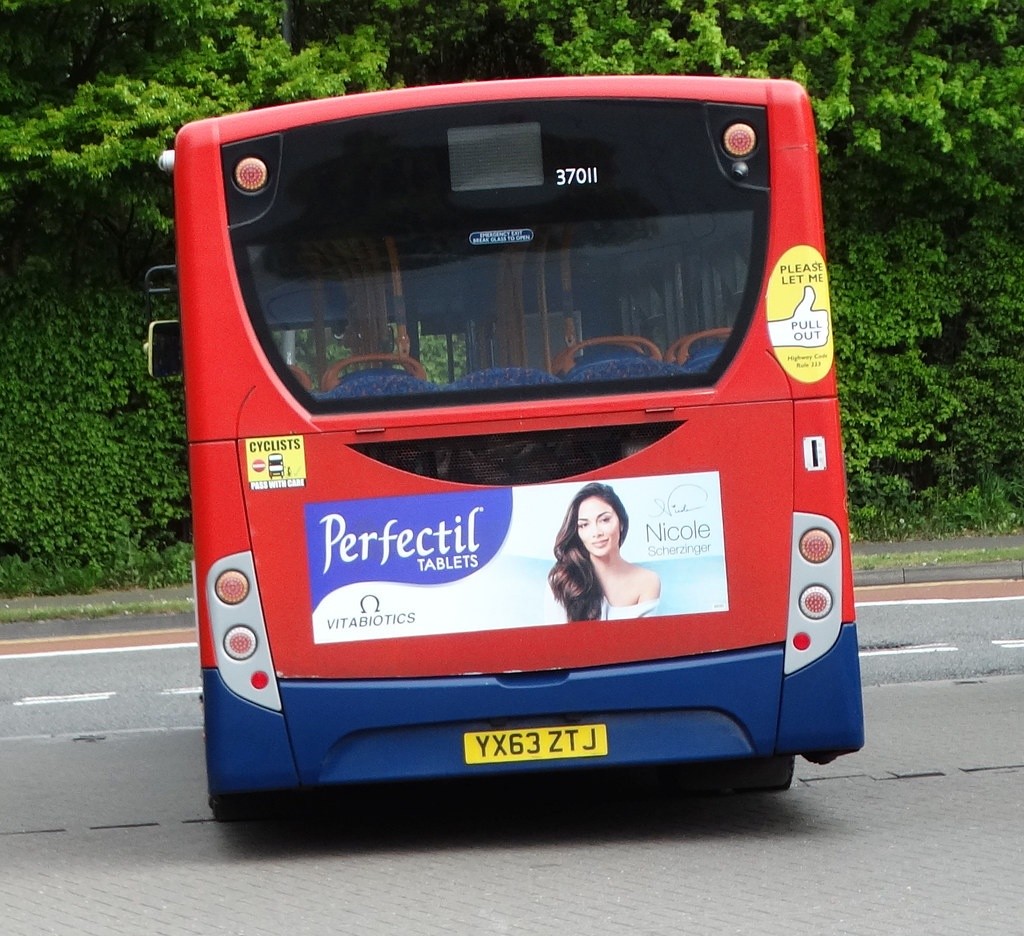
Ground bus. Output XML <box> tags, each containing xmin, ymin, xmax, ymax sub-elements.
<box><xmin>140</xmin><ymin>74</ymin><xmax>867</xmax><ymax>820</ymax></box>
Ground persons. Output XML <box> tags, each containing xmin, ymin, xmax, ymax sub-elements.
<box><xmin>548</xmin><ymin>483</ymin><xmax>661</xmax><ymax>621</ymax></box>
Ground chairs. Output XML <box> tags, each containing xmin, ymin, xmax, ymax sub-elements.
<box><xmin>285</xmin><ymin>327</ymin><xmax>734</xmax><ymax>402</ymax></box>
